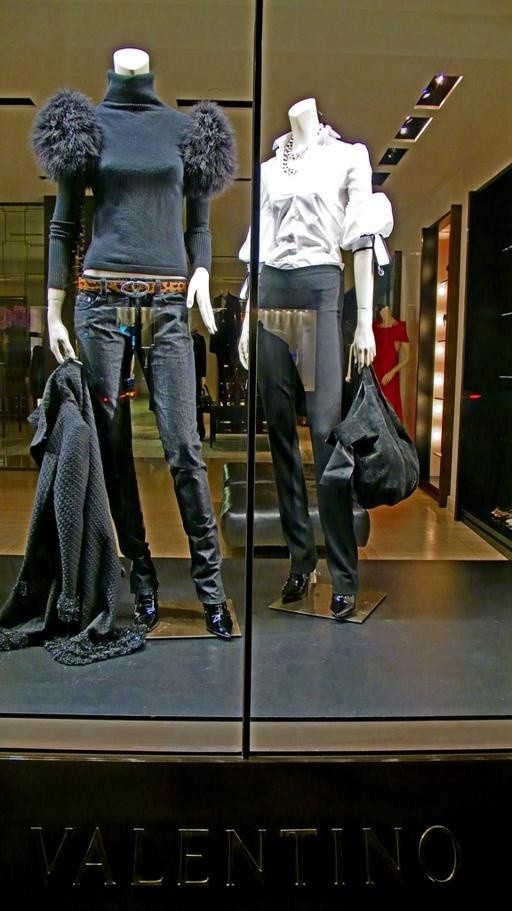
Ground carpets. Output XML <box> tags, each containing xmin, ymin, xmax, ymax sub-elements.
<box><xmin>126</xmin><ymin>399</ymin><xmax>315</xmax><ymax>461</ymax></box>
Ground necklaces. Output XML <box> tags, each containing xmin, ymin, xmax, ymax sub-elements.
<box><xmin>281</xmin><ymin>123</ymin><xmax>325</xmax><ymax>175</ymax></box>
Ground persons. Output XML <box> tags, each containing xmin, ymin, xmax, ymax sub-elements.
<box><xmin>190</xmin><ymin>329</ymin><xmax>207</xmax><ymax>440</ymax></box>
<box><xmin>237</xmin><ymin>95</ymin><xmax>393</xmax><ymax>619</ymax></box>
<box><xmin>210</xmin><ymin>288</ymin><xmax>241</xmax><ymax>403</ymax></box>
<box><xmin>370</xmin><ymin>305</ymin><xmax>410</xmax><ymax>423</ymax></box>
<box><xmin>32</xmin><ymin>48</ymin><xmax>236</xmax><ymax>639</ymax></box>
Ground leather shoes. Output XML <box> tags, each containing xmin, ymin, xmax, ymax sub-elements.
<box><xmin>205</xmin><ymin>602</ymin><xmax>232</xmax><ymax>639</ymax></box>
<box><xmin>281</xmin><ymin>572</ymin><xmax>309</xmax><ymax>603</ymax></box>
<box><xmin>134</xmin><ymin>590</ymin><xmax>159</xmax><ymax>632</ymax></box>
<box><xmin>330</xmin><ymin>594</ymin><xmax>355</xmax><ymax>618</ymax></box>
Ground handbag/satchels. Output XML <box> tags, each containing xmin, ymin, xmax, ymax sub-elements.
<box><xmin>319</xmin><ymin>364</ymin><xmax>420</xmax><ymax>508</ymax></box>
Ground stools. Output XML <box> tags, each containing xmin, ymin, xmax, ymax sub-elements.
<box><xmin>222</xmin><ymin>458</ymin><xmax>370</xmax><ymax>558</ymax></box>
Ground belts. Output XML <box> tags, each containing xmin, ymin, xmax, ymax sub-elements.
<box><xmin>78</xmin><ymin>277</ymin><xmax>187</xmax><ymax>297</ymax></box>
